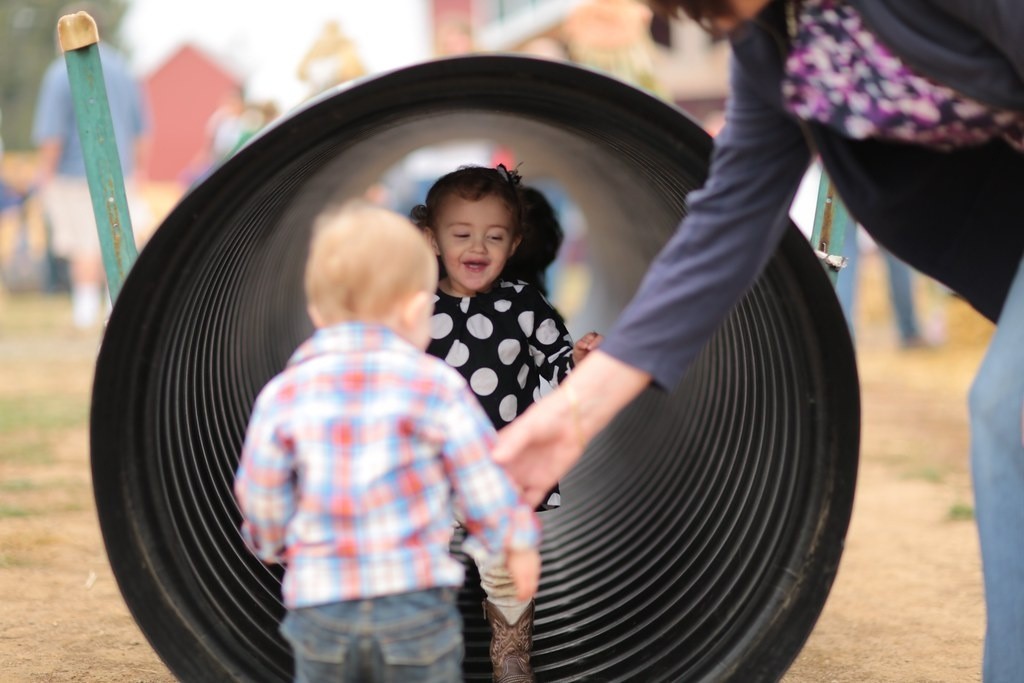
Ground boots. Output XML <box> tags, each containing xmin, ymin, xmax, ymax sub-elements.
<box><xmin>481</xmin><ymin>595</ymin><xmax>535</xmax><ymax>683</ymax></box>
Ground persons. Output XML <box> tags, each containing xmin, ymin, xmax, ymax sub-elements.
<box><xmin>30</xmin><ymin>5</ymin><xmax>148</xmax><ymax>327</ymax></box>
<box><xmin>493</xmin><ymin>0</ymin><xmax>1024</xmax><ymax>683</ymax></box>
<box><xmin>410</xmin><ymin>161</ymin><xmax>603</xmax><ymax>579</ymax></box>
<box><xmin>234</xmin><ymin>207</ymin><xmax>540</xmax><ymax>683</ymax></box>
